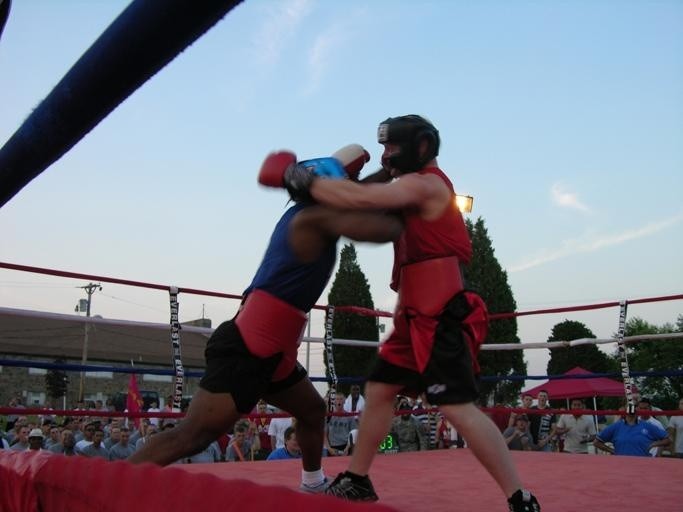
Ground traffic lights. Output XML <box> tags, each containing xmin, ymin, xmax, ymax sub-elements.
<box><xmin>45</xmin><ymin>372</ymin><xmax>69</xmax><ymax>399</ymax></box>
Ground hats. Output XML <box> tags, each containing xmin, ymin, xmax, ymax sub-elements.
<box><xmin>517</xmin><ymin>413</ymin><xmax>528</xmax><ymax>420</ymax></box>
<box><xmin>27</xmin><ymin>428</ymin><xmax>45</xmax><ymax>441</ymax></box>
<box><xmin>399</xmin><ymin>401</ymin><xmax>413</xmax><ymax>409</ymax></box>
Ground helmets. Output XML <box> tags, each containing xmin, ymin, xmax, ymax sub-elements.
<box><xmin>376</xmin><ymin>113</ymin><xmax>440</xmax><ymax>178</ymax></box>
<box><xmin>297</xmin><ymin>158</ymin><xmax>350</xmax><ymax>181</ymax></box>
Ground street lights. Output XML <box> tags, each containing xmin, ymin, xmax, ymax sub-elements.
<box><xmin>456</xmin><ymin>195</ymin><xmax>473</xmax><ymax>215</ymax></box>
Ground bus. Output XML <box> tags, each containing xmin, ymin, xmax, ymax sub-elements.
<box><xmin>113</xmin><ymin>391</ymin><xmax>159</xmax><ymax>411</ymax></box>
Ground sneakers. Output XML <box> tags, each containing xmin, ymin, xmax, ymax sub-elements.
<box><xmin>508</xmin><ymin>489</ymin><xmax>541</xmax><ymax>511</ymax></box>
<box><xmin>298</xmin><ymin>470</ymin><xmax>378</xmax><ymax>502</ymax></box>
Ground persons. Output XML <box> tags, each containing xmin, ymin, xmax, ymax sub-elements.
<box><xmin>476</xmin><ymin>392</ymin><xmax>683</xmax><ymax>458</ymax></box>
<box><xmin>260</xmin><ymin>113</ymin><xmax>540</xmax><ymax>512</ymax></box>
<box><xmin>1</xmin><ymin>392</ymin><xmax>303</xmax><ymax>465</ymax></box>
<box><xmin>114</xmin><ymin>157</ymin><xmax>400</xmax><ymax>497</ymax></box>
<box><xmin>324</xmin><ymin>392</ymin><xmax>470</xmax><ymax>457</ymax></box>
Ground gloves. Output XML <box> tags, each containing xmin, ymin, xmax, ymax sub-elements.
<box><xmin>256</xmin><ymin>151</ymin><xmax>318</xmax><ymax>204</ymax></box>
<box><xmin>329</xmin><ymin>144</ymin><xmax>371</xmax><ymax>182</ymax></box>
